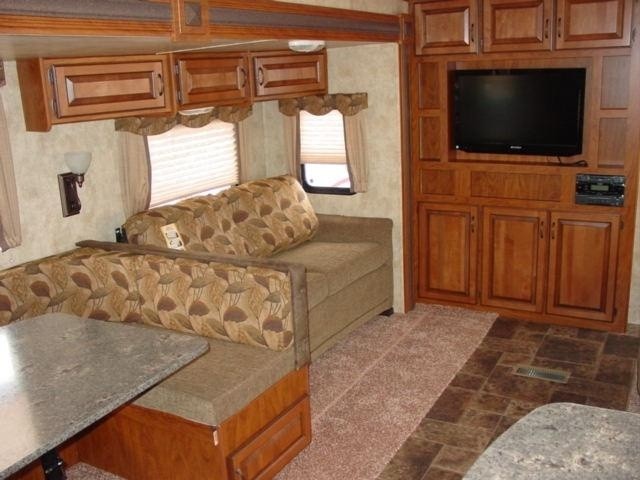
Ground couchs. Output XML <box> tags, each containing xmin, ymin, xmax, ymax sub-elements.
<box><xmin>1</xmin><ymin>240</ymin><xmax>312</xmax><ymax>480</ymax></box>
<box><xmin>121</xmin><ymin>174</ymin><xmax>394</xmax><ymax>364</ymax></box>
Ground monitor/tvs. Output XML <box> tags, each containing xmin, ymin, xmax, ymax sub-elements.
<box><xmin>453</xmin><ymin>67</ymin><xmax>585</xmax><ymax>156</ymax></box>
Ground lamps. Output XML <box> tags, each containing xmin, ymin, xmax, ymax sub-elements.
<box><xmin>56</xmin><ymin>151</ymin><xmax>92</xmax><ymax>217</ymax></box>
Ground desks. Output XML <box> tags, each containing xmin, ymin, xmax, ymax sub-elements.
<box><xmin>1</xmin><ymin>311</ymin><xmax>211</xmax><ymax>480</ymax></box>
<box><xmin>461</xmin><ymin>402</ymin><xmax>640</xmax><ymax>479</ymax></box>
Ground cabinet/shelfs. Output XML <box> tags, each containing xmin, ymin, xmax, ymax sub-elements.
<box><xmin>170</xmin><ymin>49</ymin><xmax>252</xmax><ymax>110</ymax></box>
<box><xmin>15</xmin><ymin>52</ymin><xmax>174</xmax><ymax>132</ymax></box>
<box><xmin>251</xmin><ymin>48</ymin><xmax>327</xmax><ymax>103</ymax></box>
<box><xmin>406</xmin><ymin>2</ymin><xmax>640</xmax><ymax>333</ymax></box>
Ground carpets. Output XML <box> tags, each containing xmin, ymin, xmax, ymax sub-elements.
<box><xmin>66</xmin><ymin>302</ymin><xmax>499</xmax><ymax>480</ymax></box>
